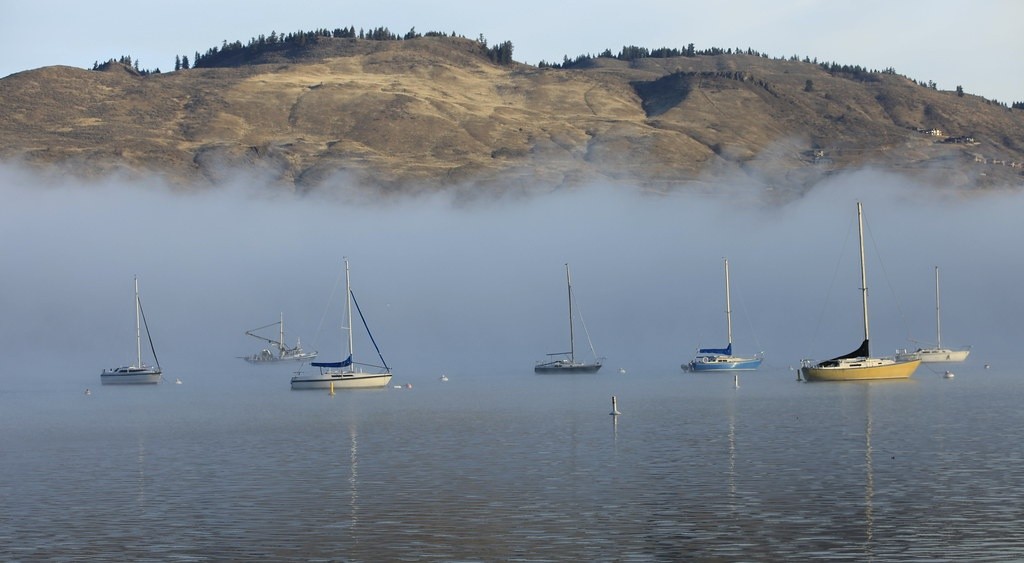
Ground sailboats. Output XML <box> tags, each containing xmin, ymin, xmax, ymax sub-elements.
<box><xmin>800</xmin><ymin>201</ymin><xmax>924</xmax><ymax>380</ymax></box>
<box><xmin>534</xmin><ymin>262</ymin><xmax>605</xmax><ymax>375</ymax></box>
<box><xmin>236</xmin><ymin>311</ymin><xmax>318</xmax><ymax>365</ymax></box>
<box><xmin>895</xmin><ymin>266</ymin><xmax>973</xmax><ymax>361</ymax></box>
<box><xmin>100</xmin><ymin>273</ymin><xmax>164</xmax><ymax>385</ymax></box>
<box><xmin>680</xmin><ymin>258</ymin><xmax>765</xmax><ymax>373</ymax></box>
<box><xmin>289</xmin><ymin>256</ymin><xmax>392</xmax><ymax>390</ymax></box>
